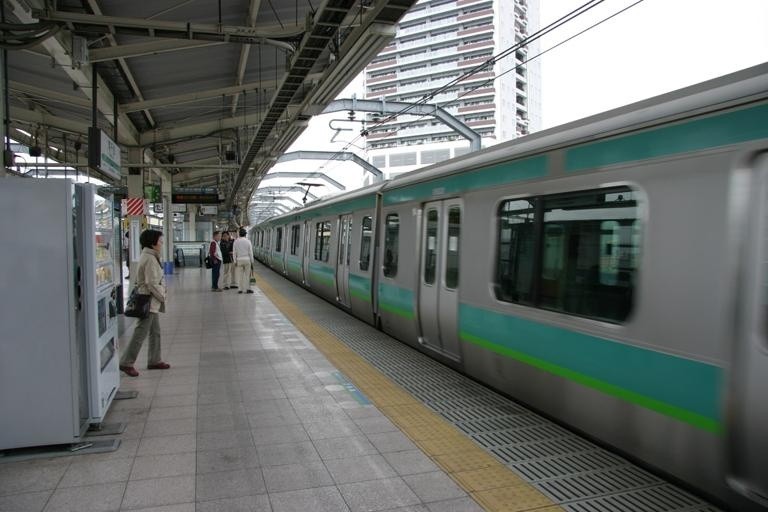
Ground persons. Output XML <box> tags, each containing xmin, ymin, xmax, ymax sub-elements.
<box><xmin>119</xmin><ymin>230</ymin><xmax>170</xmax><ymax>377</ymax></box>
<box><xmin>123</xmin><ymin>232</ymin><xmax>130</xmax><ymax>279</ymax></box>
<box><xmin>205</xmin><ymin>229</ymin><xmax>254</xmax><ymax>293</ymax></box>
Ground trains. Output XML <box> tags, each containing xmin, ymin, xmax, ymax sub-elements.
<box><xmin>247</xmin><ymin>68</ymin><xmax>768</xmax><ymax>512</ymax></box>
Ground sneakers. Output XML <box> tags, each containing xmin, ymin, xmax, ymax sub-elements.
<box><xmin>211</xmin><ymin>285</ymin><xmax>254</xmax><ymax>294</ymax></box>
<box><xmin>146</xmin><ymin>362</ymin><xmax>170</xmax><ymax>370</ymax></box>
<box><xmin>119</xmin><ymin>364</ymin><xmax>140</xmax><ymax>376</ymax></box>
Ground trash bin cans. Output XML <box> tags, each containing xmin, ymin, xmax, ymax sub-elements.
<box><xmin>164</xmin><ymin>262</ymin><xmax>173</xmax><ymax>274</ymax></box>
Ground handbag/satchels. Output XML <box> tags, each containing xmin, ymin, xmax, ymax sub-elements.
<box><xmin>123</xmin><ymin>292</ymin><xmax>152</xmax><ymax>318</ymax></box>
<box><xmin>204</xmin><ymin>256</ymin><xmax>215</xmax><ymax>269</ymax></box>
